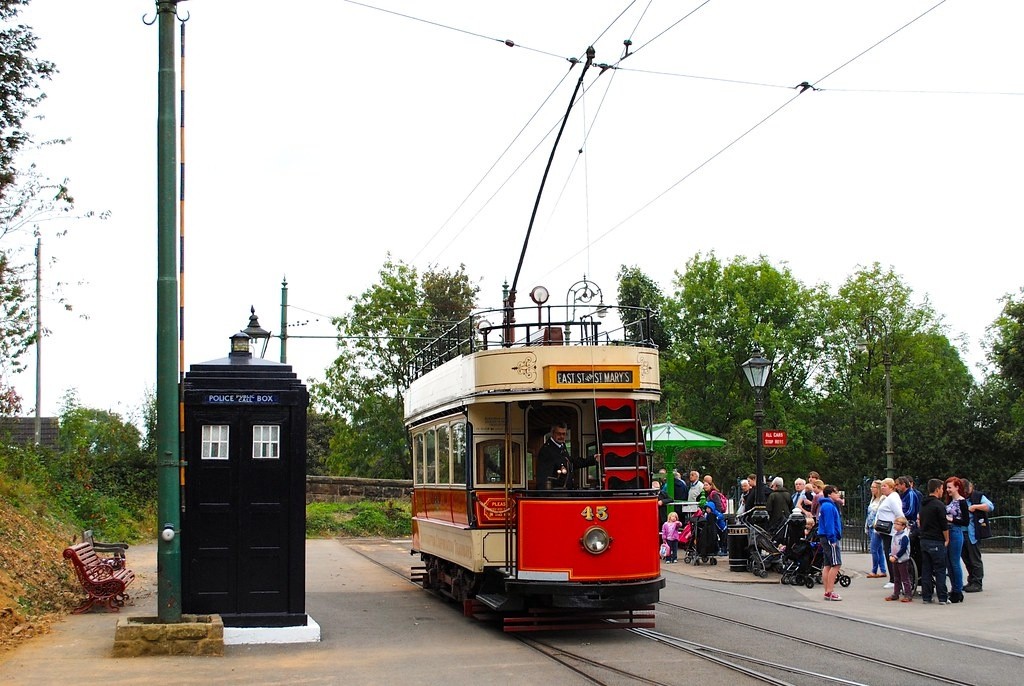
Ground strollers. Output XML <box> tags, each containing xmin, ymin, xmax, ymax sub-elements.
<box><xmin>740</xmin><ymin>507</ymin><xmax>792</xmax><ymax>578</ymax></box>
<box><xmin>684</xmin><ymin>511</ymin><xmax>720</xmax><ymax>566</ymax></box>
<box><xmin>780</xmin><ymin>523</ymin><xmax>850</xmax><ymax>588</ymax></box>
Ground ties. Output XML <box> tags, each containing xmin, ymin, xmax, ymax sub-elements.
<box><xmin>793</xmin><ymin>493</ymin><xmax>800</xmax><ymax>506</ymax></box>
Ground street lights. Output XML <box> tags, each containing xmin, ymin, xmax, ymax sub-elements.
<box><xmin>741</xmin><ymin>342</ymin><xmax>773</xmax><ymax>530</ymax></box>
<box><xmin>855</xmin><ymin>313</ymin><xmax>898</xmax><ymax>480</ymax></box>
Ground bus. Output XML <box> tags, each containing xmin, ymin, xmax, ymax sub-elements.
<box><xmin>396</xmin><ymin>44</ymin><xmax>667</xmax><ymax>634</ymax></box>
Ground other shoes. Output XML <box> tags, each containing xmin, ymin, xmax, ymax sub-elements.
<box><xmin>884</xmin><ymin>582</ymin><xmax>894</xmax><ymax>589</ymax></box>
<box><xmin>922</xmin><ymin>598</ymin><xmax>935</xmax><ymax>604</ymax></box>
<box><xmin>666</xmin><ymin>560</ymin><xmax>671</xmax><ymax>564</ymax></box>
<box><xmin>866</xmin><ymin>573</ymin><xmax>887</xmax><ymax>578</ymax></box>
<box><xmin>962</xmin><ymin>582</ymin><xmax>983</xmax><ymax>592</ymax></box>
<box><xmin>673</xmin><ymin>560</ymin><xmax>678</xmax><ymax>563</ymax></box>
<box><xmin>885</xmin><ymin>594</ymin><xmax>899</xmax><ymax>601</ymax></box>
<box><xmin>939</xmin><ymin>599</ymin><xmax>951</xmax><ymax>604</ymax></box>
<box><xmin>901</xmin><ymin>595</ymin><xmax>912</xmax><ymax>602</ymax></box>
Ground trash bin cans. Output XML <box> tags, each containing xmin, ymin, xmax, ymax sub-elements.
<box><xmin>728</xmin><ymin>525</ymin><xmax>749</xmax><ymax>571</ymax></box>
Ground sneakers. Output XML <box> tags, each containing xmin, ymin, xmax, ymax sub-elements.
<box><xmin>824</xmin><ymin>591</ymin><xmax>842</xmax><ymax>602</ymax></box>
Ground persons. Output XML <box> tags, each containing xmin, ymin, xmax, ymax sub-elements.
<box><xmin>736</xmin><ymin>470</ymin><xmax>843</xmax><ymax>530</ymax></box>
<box><xmin>864</xmin><ymin>477</ymin><xmax>994</xmax><ymax>605</ymax></box>
<box><xmin>653</xmin><ymin>469</ymin><xmax>727</xmax><ymax>564</ymax></box>
<box><xmin>535</xmin><ymin>422</ymin><xmax>600</xmax><ymax>489</ymax></box>
<box><xmin>817</xmin><ymin>485</ymin><xmax>843</xmax><ymax>601</ymax></box>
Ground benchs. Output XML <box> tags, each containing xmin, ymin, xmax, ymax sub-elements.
<box><xmin>82</xmin><ymin>529</ymin><xmax>129</xmax><ymax>571</ymax></box>
<box><xmin>63</xmin><ymin>541</ymin><xmax>136</xmax><ymax>614</ymax></box>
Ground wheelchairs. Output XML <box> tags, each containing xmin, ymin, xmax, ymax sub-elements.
<box><xmin>898</xmin><ymin>551</ymin><xmax>950</xmax><ymax>599</ymax></box>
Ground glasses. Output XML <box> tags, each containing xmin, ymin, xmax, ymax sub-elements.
<box><xmin>894</xmin><ymin>484</ymin><xmax>899</xmax><ymax>487</ymax></box>
<box><xmin>870</xmin><ymin>486</ymin><xmax>877</xmax><ymax>489</ymax></box>
<box><xmin>554</xmin><ymin>431</ymin><xmax>566</xmax><ymax>436</ymax></box>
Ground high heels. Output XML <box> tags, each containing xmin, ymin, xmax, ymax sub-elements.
<box><xmin>947</xmin><ymin>590</ymin><xmax>964</xmax><ymax>603</ymax></box>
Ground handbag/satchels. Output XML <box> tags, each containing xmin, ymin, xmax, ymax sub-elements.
<box><xmin>874</xmin><ymin>519</ymin><xmax>893</xmax><ymax>534</ymax></box>
<box><xmin>660</xmin><ymin>542</ymin><xmax>671</xmax><ymax>558</ymax></box>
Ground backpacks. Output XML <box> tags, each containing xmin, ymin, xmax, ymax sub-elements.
<box><xmin>709</xmin><ymin>490</ymin><xmax>727</xmax><ymax>513</ymax></box>
